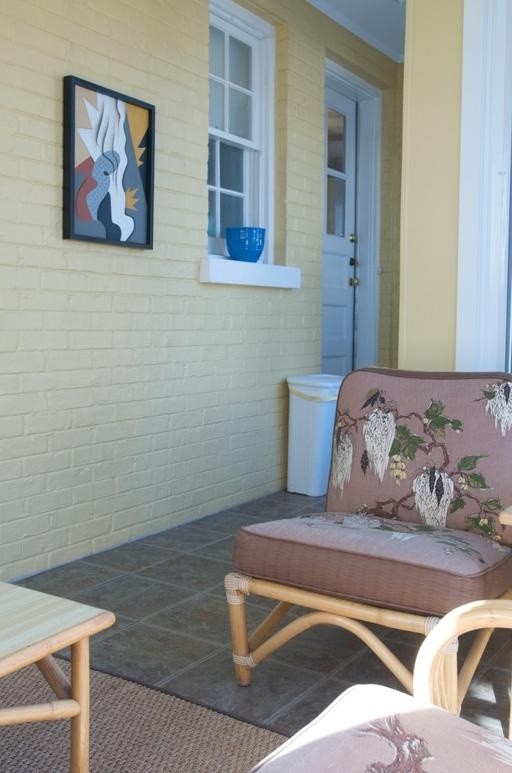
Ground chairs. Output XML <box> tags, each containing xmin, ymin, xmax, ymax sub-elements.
<box><xmin>258</xmin><ymin>600</ymin><xmax>512</xmax><ymax>773</ymax></box>
<box><xmin>226</xmin><ymin>367</ymin><xmax>511</xmax><ymax>710</ymax></box>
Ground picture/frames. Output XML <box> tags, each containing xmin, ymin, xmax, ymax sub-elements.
<box><xmin>63</xmin><ymin>75</ymin><xmax>154</xmax><ymax>249</ymax></box>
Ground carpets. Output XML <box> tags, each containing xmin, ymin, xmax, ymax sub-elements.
<box><xmin>0</xmin><ymin>654</ymin><xmax>290</xmax><ymax>773</ymax></box>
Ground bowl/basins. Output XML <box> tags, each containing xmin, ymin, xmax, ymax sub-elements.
<box><xmin>226</xmin><ymin>227</ymin><xmax>265</xmax><ymax>262</ymax></box>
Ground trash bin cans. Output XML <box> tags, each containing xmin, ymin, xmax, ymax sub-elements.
<box><xmin>286</xmin><ymin>374</ymin><xmax>346</xmax><ymax>497</ymax></box>
